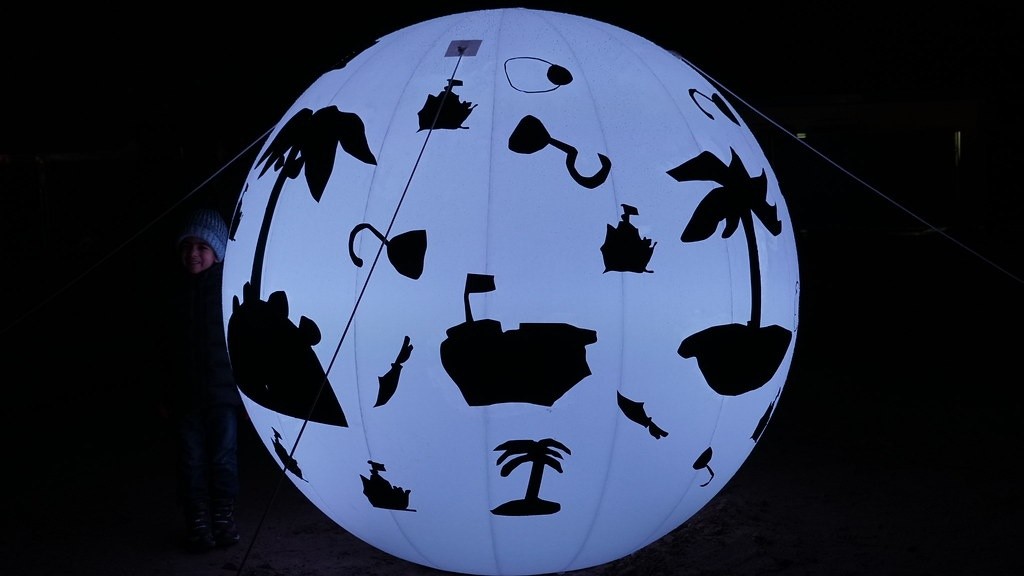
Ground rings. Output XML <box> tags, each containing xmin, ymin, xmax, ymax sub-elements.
<box><xmin>503</xmin><ymin>56</ymin><xmax>572</xmax><ymax>94</ymax></box>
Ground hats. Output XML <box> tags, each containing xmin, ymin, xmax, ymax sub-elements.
<box><xmin>177</xmin><ymin>209</ymin><xmax>229</xmax><ymax>263</ymax></box>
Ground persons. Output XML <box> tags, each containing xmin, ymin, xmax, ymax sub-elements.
<box><xmin>155</xmin><ymin>205</ymin><xmax>258</xmax><ymax>551</ymax></box>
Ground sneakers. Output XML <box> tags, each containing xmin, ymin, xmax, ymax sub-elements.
<box><xmin>211</xmin><ymin>502</ymin><xmax>241</xmax><ymax>544</ymax></box>
<box><xmin>189</xmin><ymin>500</ymin><xmax>215</xmax><ymax>546</ymax></box>
